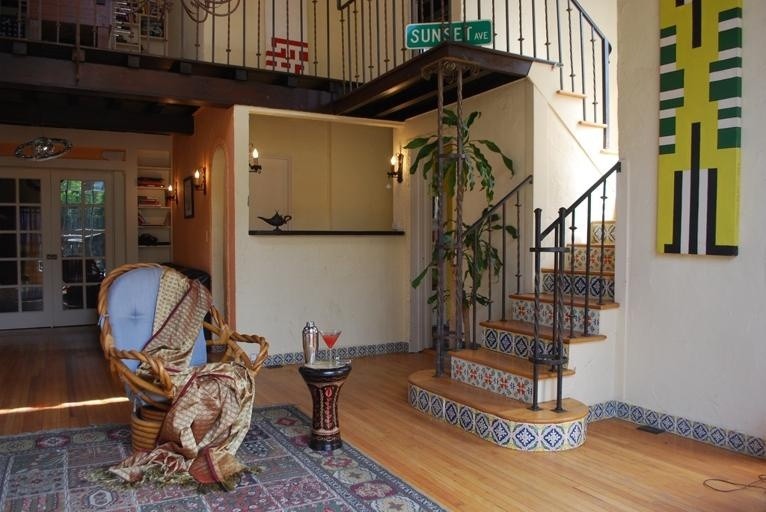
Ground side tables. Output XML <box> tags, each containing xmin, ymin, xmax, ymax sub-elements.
<box><xmin>298</xmin><ymin>361</ymin><xmax>352</xmax><ymax>451</ymax></box>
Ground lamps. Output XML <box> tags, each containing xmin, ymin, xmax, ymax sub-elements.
<box><xmin>249</xmin><ymin>148</ymin><xmax>262</xmax><ymax>172</ymax></box>
<box><xmin>386</xmin><ymin>153</ymin><xmax>404</xmax><ymax>183</ymax></box>
<box><xmin>193</xmin><ymin>168</ymin><xmax>207</xmax><ymax>195</ymax></box>
<box><xmin>166</xmin><ymin>181</ymin><xmax>177</xmax><ymax>205</ymax></box>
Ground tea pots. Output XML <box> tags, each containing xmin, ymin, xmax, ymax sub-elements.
<box><xmin>256</xmin><ymin>212</ymin><xmax>291</xmax><ymax>226</ymax></box>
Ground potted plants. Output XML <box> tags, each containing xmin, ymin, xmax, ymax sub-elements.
<box><xmin>404</xmin><ymin>108</ymin><xmax>519</xmax><ymax>376</ymax></box>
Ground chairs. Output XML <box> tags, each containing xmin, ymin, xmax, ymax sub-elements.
<box><xmin>97</xmin><ymin>262</ymin><xmax>269</xmax><ymax>455</ymax></box>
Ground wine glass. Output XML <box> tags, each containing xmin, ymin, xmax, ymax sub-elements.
<box><xmin>319</xmin><ymin>329</ymin><xmax>341</xmax><ymax>367</ymax></box>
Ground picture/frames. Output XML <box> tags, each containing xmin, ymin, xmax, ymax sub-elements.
<box><xmin>182</xmin><ymin>175</ymin><xmax>194</xmax><ymax>219</ymax></box>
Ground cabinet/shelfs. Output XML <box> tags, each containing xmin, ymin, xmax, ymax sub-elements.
<box><xmin>113</xmin><ymin>0</ymin><xmax>168</xmax><ymax>57</ymax></box>
<box><xmin>135</xmin><ymin>148</ymin><xmax>173</xmax><ymax>265</ymax></box>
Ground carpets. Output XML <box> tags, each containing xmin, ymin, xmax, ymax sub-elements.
<box><xmin>0</xmin><ymin>402</ymin><xmax>444</xmax><ymax>512</ymax></box>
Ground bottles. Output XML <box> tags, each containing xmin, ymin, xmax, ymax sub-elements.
<box><xmin>302</xmin><ymin>320</ymin><xmax>319</xmax><ymax>365</ymax></box>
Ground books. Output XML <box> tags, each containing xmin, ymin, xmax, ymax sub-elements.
<box><xmin>137</xmin><ymin>175</ymin><xmax>167</xmax><ymax>208</ymax></box>
